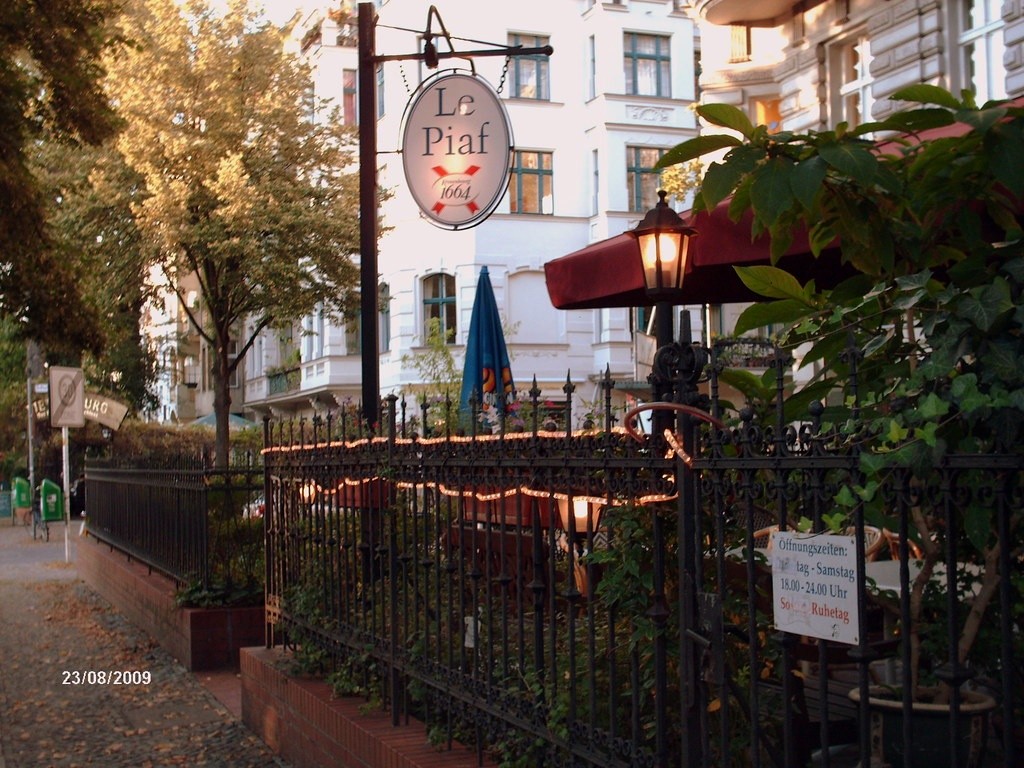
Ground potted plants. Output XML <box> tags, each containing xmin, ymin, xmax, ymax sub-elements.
<box><xmin>658</xmin><ymin>80</ymin><xmax>1024</xmax><ymax>768</ymax></box>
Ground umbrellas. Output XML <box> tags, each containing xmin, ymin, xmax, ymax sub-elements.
<box><xmin>459</xmin><ymin>265</ymin><xmax>520</xmax><ymax>431</ymax></box>
<box><xmin>542</xmin><ymin>94</ymin><xmax>1024</xmax><ymax>308</ymax></box>
<box><xmin>193</xmin><ymin>412</ymin><xmax>255</xmax><ymax>429</ymax></box>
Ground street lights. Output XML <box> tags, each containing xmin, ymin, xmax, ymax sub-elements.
<box><xmin>623</xmin><ymin>190</ymin><xmax>696</xmax><ymax>458</ymax></box>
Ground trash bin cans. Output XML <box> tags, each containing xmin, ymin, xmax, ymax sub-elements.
<box><xmin>39</xmin><ymin>478</ymin><xmax>63</xmax><ymax>521</ymax></box>
<box><xmin>10</xmin><ymin>476</ymin><xmax>30</xmax><ymax>507</ymax></box>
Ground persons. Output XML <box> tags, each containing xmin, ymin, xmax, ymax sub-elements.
<box><xmin>59</xmin><ymin>473</ymin><xmax>85</xmax><ymax>518</ymax></box>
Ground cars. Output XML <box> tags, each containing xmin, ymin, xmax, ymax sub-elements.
<box><xmin>243</xmin><ymin>494</ymin><xmax>268</xmax><ymax>519</ymax></box>
